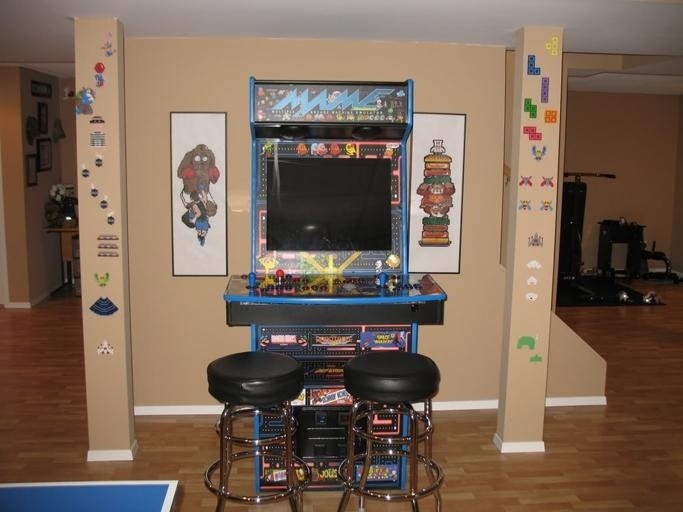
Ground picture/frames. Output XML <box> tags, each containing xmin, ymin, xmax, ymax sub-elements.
<box><xmin>404</xmin><ymin>111</ymin><xmax>467</xmax><ymax>275</ymax></box>
<box><xmin>168</xmin><ymin>110</ymin><xmax>229</xmax><ymax>278</ymax></box>
<box><xmin>25</xmin><ymin>76</ymin><xmax>56</xmax><ymax>188</ymax></box>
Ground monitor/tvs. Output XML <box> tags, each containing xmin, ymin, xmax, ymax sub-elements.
<box><xmin>257</xmin><ymin>142</ymin><xmax>402</xmax><ymax>276</ymax></box>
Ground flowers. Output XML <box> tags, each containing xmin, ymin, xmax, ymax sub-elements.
<box><xmin>42</xmin><ymin>179</ymin><xmax>77</xmax><ymax>226</ymax></box>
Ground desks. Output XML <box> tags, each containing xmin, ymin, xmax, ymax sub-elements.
<box><xmin>41</xmin><ymin>219</ymin><xmax>80</xmax><ymax>301</ymax></box>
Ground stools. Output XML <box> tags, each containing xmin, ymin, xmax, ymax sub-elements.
<box><xmin>200</xmin><ymin>350</ymin><xmax>312</xmax><ymax>511</ymax></box>
<box><xmin>333</xmin><ymin>351</ymin><xmax>445</xmax><ymax>511</ymax></box>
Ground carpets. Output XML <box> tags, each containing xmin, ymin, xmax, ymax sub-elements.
<box><xmin>555</xmin><ymin>280</ymin><xmax>667</xmax><ymax>308</ymax></box>
